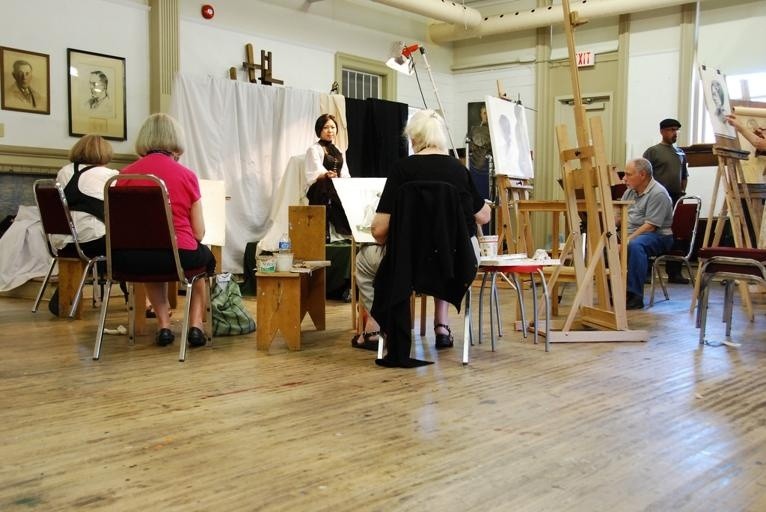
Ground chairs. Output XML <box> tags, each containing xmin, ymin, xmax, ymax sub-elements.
<box><xmin>647</xmin><ymin>194</ymin><xmax>765</xmax><ymax>345</ymax></box>
<box><xmin>32</xmin><ymin>176</ymin><xmax>106</xmax><ymax>321</ymax></box>
<box><xmin>90</xmin><ymin>172</ymin><xmax>214</xmax><ymax>361</ymax></box>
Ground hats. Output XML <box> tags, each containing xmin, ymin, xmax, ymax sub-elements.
<box><xmin>659</xmin><ymin>119</ymin><xmax>680</xmax><ymax>129</ymax></box>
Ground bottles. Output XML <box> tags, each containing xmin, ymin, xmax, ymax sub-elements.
<box><xmin>279</xmin><ymin>231</ymin><xmax>291</xmax><ymax>272</ymax></box>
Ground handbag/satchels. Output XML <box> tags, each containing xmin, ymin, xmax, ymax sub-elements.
<box><xmin>210</xmin><ymin>271</ymin><xmax>257</xmax><ymax>338</ymax></box>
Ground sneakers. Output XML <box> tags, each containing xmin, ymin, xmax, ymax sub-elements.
<box><xmin>625</xmin><ymin>295</ymin><xmax>645</xmax><ymax>310</ymax></box>
<box><xmin>643</xmin><ymin>266</ymin><xmax>653</xmax><ymax>285</ymax></box>
<box><xmin>667</xmin><ymin>273</ymin><xmax>690</xmax><ymax>284</ymax></box>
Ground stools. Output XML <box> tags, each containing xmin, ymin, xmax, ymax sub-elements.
<box><xmin>477</xmin><ymin>263</ymin><xmax>552</xmax><ymax>353</ymax></box>
<box><xmin>377</xmin><ymin>280</ymin><xmax>475</xmax><ymax>364</ymax></box>
<box><xmin>254</xmin><ymin>204</ymin><xmax>333</xmax><ymax>353</ymax></box>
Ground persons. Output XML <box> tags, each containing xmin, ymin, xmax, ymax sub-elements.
<box><xmin>303</xmin><ymin>112</ymin><xmax>351</xmax><ymax>244</ymax></box>
<box><xmin>7</xmin><ymin>59</ymin><xmax>47</xmax><ymax>113</ymax></box>
<box><xmin>80</xmin><ymin>70</ymin><xmax>116</xmax><ymax>118</ymax></box>
<box><xmin>351</xmin><ymin>109</ymin><xmax>491</xmax><ymax>351</ymax></box>
<box><xmin>724</xmin><ymin>112</ymin><xmax>766</xmax><ymax>158</ymax></box>
<box><xmin>642</xmin><ymin>117</ymin><xmax>691</xmax><ymax>284</ymax></box>
<box><xmin>711</xmin><ymin>81</ymin><xmax>727</xmax><ymax>125</ymax></box>
<box><xmin>113</xmin><ymin>113</ymin><xmax>217</xmax><ymax>348</ymax></box>
<box><xmin>603</xmin><ymin>157</ymin><xmax>676</xmax><ymax>310</ymax></box>
<box><xmin>45</xmin><ymin>133</ymin><xmax>128</xmax><ymax>316</ymax></box>
<box><xmin>480</xmin><ymin>105</ymin><xmax>488</xmax><ymax>127</ymax></box>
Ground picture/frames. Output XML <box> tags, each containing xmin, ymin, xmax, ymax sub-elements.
<box><xmin>66</xmin><ymin>47</ymin><xmax>129</xmax><ymax>142</ymax></box>
<box><xmin>0</xmin><ymin>45</ymin><xmax>52</xmax><ymax>116</ymax></box>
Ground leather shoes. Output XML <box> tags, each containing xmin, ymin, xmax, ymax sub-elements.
<box><xmin>188</xmin><ymin>327</ymin><xmax>206</xmax><ymax>347</ymax></box>
<box><xmin>157</xmin><ymin>328</ymin><xmax>175</xmax><ymax>346</ymax></box>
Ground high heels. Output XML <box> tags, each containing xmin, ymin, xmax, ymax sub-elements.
<box><xmin>433</xmin><ymin>323</ymin><xmax>453</xmax><ymax>349</ymax></box>
<box><xmin>351</xmin><ymin>331</ymin><xmax>381</xmax><ymax>350</ymax></box>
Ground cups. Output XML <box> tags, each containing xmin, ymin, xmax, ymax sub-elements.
<box><xmin>274</xmin><ymin>253</ymin><xmax>295</xmax><ymax>271</ymax></box>
<box><xmin>480</xmin><ymin>235</ymin><xmax>498</xmax><ymax>259</ymax></box>
<box><xmin>257</xmin><ymin>255</ymin><xmax>274</xmax><ymax>272</ymax></box>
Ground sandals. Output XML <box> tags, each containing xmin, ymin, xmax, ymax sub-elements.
<box><xmin>144</xmin><ymin>305</ymin><xmax>173</xmax><ymax>319</ymax></box>
